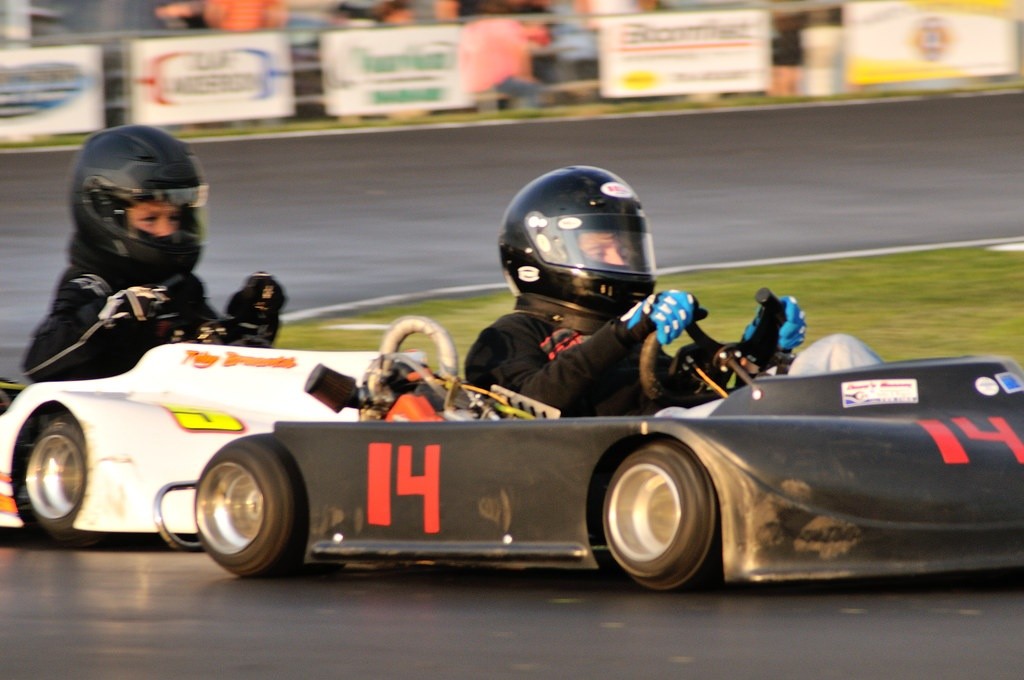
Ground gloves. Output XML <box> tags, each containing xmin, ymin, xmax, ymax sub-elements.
<box><xmin>225</xmin><ymin>272</ymin><xmax>289</xmax><ymax>321</ymax></box>
<box><xmin>97</xmin><ymin>283</ymin><xmax>169</xmax><ymax>328</ymax></box>
<box><xmin>745</xmin><ymin>296</ymin><xmax>806</xmax><ymax>357</ymax></box>
<box><xmin>630</xmin><ymin>288</ymin><xmax>709</xmax><ymax>345</ymax></box>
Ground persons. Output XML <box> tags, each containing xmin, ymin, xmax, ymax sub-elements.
<box><xmin>464</xmin><ymin>164</ymin><xmax>883</xmax><ymax>420</ymax></box>
<box><xmin>20</xmin><ymin>124</ymin><xmax>290</xmax><ymax>384</ymax></box>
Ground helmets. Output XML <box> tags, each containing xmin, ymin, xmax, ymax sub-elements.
<box><xmin>72</xmin><ymin>125</ymin><xmax>209</xmax><ymax>275</ymax></box>
<box><xmin>498</xmin><ymin>165</ymin><xmax>659</xmax><ymax>320</ymax></box>
